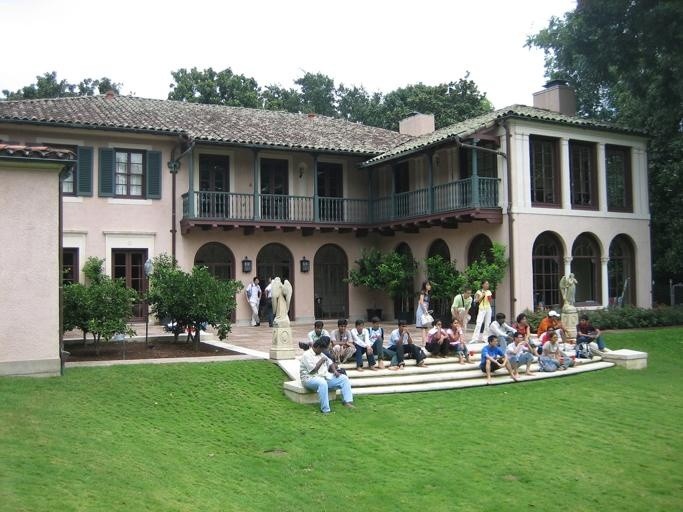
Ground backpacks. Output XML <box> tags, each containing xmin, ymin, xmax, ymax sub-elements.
<box><xmin>576</xmin><ymin>342</ymin><xmax>594</xmax><ymax>360</ymax></box>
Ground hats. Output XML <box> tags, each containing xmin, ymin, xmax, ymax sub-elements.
<box><xmin>547</xmin><ymin>310</ymin><xmax>560</xmax><ymax>318</ymax></box>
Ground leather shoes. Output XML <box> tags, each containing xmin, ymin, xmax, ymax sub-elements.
<box><xmin>356</xmin><ymin>360</ymin><xmax>429</xmax><ymax>372</ymax></box>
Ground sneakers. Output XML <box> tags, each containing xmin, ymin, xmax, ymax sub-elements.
<box><xmin>469</xmin><ymin>338</ymin><xmax>488</xmax><ymax>344</ymax></box>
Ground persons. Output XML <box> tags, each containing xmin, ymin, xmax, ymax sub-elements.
<box><xmin>307</xmin><ymin>317</ymin><xmax>428</xmax><ymax>378</ymax></box>
<box><xmin>414</xmin><ymin>280</ymin><xmax>433</xmax><ymax>348</ymax></box>
<box><xmin>566</xmin><ymin>273</ymin><xmax>578</xmax><ymax>306</ymax></box>
<box><xmin>450</xmin><ymin>285</ymin><xmax>473</xmax><ymax>344</ymax></box>
<box><xmin>264</xmin><ymin>275</ymin><xmax>276</xmax><ymax>327</ymax></box>
<box><xmin>446</xmin><ymin>318</ymin><xmax>474</xmax><ymax>364</ymax></box>
<box><xmin>299</xmin><ymin>339</ymin><xmax>355</xmax><ymax>414</ymax></box>
<box><xmin>424</xmin><ymin>319</ymin><xmax>449</xmax><ymax>358</ymax></box>
<box><xmin>245</xmin><ymin>276</ymin><xmax>262</xmax><ymax>327</ymax></box>
<box><xmin>478</xmin><ymin>310</ymin><xmax>606</xmax><ymax>384</ymax></box>
<box><xmin>467</xmin><ymin>279</ymin><xmax>494</xmax><ymax>344</ymax></box>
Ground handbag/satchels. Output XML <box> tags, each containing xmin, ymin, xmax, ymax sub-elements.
<box><xmin>419</xmin><ymin>313</ymin><xmax>435</xmax><ymax>326</ymax></box>
<box><xmin>246</xmin><ymin>289</ymin><xmax>252</xmax><ymax>298</ymax></box>
<box><xmin>536</xmin><ymin>355</ymin><xmax>557</xmax><ymax>373</ymax></box>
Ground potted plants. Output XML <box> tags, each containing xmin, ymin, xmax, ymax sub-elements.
<box><xmin>340</xmin><ymin>245</ymin><xmax>388</xmax><ymax>323</ymax></box>
<box><xmin>373</xmin><ymin>249</ymin><xmax>420</xmax><ymax>320</ymax></box>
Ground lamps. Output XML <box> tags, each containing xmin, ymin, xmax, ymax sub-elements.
<box><xmin>241</xmin><ymin>256</ymin><xmax>251</xmax><ymax>273</ymax></box>
<box><xmin>299</xmin><ymin>255</ymin><xmax>310</xmax><ymax>274</ymax></box>
<box><xmin>295</xmin><ymin>161</ymin><xmax>308</xmax><ymax>180</ymax></box>
<box><xmin>431</xmin><ymin>153</ymin><xmax>440</xmax><ymax>168</ymax></box>
